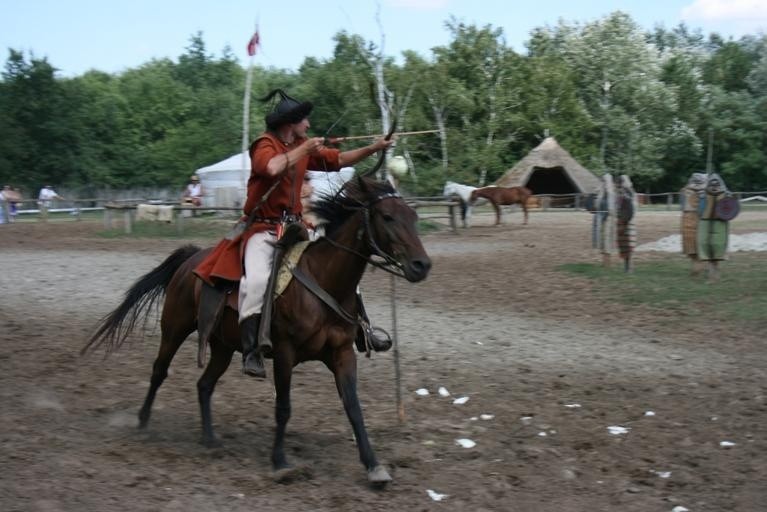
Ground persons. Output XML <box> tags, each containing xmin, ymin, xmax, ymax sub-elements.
<box><xmin>238</xmin><ymin>89</ymin><xmax>394</xmax><ymax>379</ymax></box>
<box><xmin>187</xmin><ymin>176</ymin><xmax>205</xmax><ymax>206</ymax></box>
<box><xmin>0</xmin><ymin>185</ymin><xmax>24</xmax><ymax>224</ymax></box>
<box><xmin>592</xmin><ymin>173</ymin><xmax>635</xmax><ymax>272</ymax></box>
<box><xmin>37</xmin><ymin>186</ymin><xmax>64</xmax><ymax>218</ymax></box>
<box><xmin>681</xmin><ymin>172</ymin><xmax>739</xmax><ymax>261</ymax></box>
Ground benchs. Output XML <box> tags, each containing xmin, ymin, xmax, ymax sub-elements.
<box><xmin>248</xmin><ymin>32</ymin><xmax>259</xmax><ymax>55</ymax></box>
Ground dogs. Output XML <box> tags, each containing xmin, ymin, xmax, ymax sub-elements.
<box><xmin>470</xmin><ymin>186</ymin><xmax>532</xmax><ymax>226</ymax></box>
<box><xmin>441</xmin><ymin>180</ymin><xmax>505</xmax><ymax>228</ymax></box>
<box><xmin>78</xmin><ymin>172</ymin><xmax>431</xmax><ymax>488</ymax></box>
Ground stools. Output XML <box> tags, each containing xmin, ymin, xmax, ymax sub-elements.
<box><xmin>267</xmin><ymin>215</ymin><xmax>297</xmax><ymax>223</ymax></box>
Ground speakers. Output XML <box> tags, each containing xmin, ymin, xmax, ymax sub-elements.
<box><xmin>241</xmin><ymin>313</ymin><xmax>267</xmax><ymax>378</ymax></box>
<box><xmin>355</xmin><ymin>294</ymin><xmax>393</xmax><ymax>352</ymax></box>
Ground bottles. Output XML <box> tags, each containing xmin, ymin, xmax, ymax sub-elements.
<box><xmin>252</xmin><ymin>89</ymin><xmax>314</xmax><ymax>128</ymax></box>
